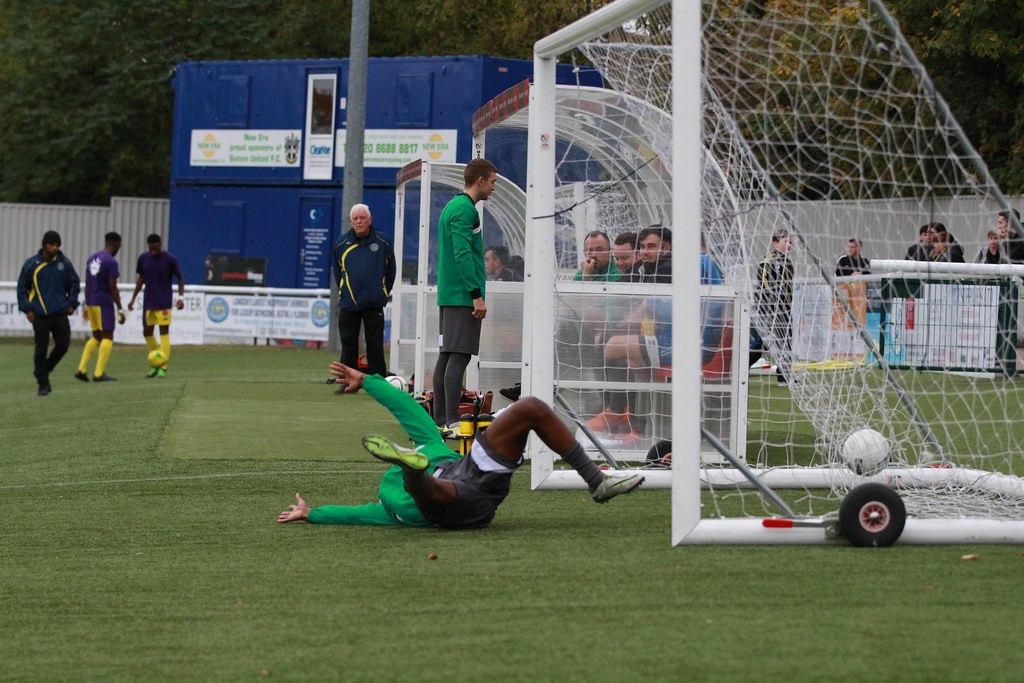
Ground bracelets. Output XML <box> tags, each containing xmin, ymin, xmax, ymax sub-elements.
<box><xmin>118</xmin><ymin>309</ymin><xmax>123</xmax><ymax>313</ymax></box>
<box><xmin>178</xmin><ymin>295</ymin><xmax>183</xmax><ymax>300</ymax></box>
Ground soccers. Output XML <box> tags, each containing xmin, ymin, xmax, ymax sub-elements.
<box><xmin>841</xmin><ymin>429</ymin><xmax>890</xmax><ymax>476</ymax></box>
<box><xmin>384</xmin><ymin>376</ymin><xmax>408</xmax><ymax>394</ymax></box>
<box><xmin>147</xmin><ymin>350</ymin><xmax>167</xmax><ymax>367</ymax></box>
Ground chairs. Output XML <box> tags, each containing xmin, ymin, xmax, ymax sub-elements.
<box><xmin>651</xmin><ymin>319</ymin><xmax>734</xmax><ymax>440</ymax></box>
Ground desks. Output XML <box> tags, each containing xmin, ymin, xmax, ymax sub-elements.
<box><xmin>879</xmin><ymin>275</ymin><xmax>1018</xmax><ymax>378</ymax></box>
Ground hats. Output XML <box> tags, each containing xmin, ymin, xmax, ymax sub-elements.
<box><xmin>42</xmin><ymin>230</ymin><xmax>60</xmax><ymax>247</ymax></box>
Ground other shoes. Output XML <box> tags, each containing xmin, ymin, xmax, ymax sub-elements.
<box><xmin>38</xmin><ymin>385</ymin><xmax>49</xmax><ymax>395</ymax></box>
<box><xmin>33</xmin><ymin>369</ymin><xmax>51</xmax><ymax>392</ymax></box>
<box><xmin>335</xmin><ymin>384</ymin><xmax>357</xmax><ymax>394</ymax></box>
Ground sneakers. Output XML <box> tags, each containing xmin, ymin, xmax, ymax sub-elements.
<box><xmin>590</xmin><ymin>473</ymin><xmax>646</xmax><ymax>505</ymax></box>
<box><xmin>74</xmin><ymin>370</ymin><xmax>90</xmax><ymax>382</ymax></box>
<box><xmin>616</xmin><ymin>431</ymin><xmax>645</xmax><ymax>447</ymax></box>
<box><xmin>586</xmin><ymin>405</ymin><xmax>631</xmax><ymax>430</ymax></box>
<box><xmin>156</xmin><ymin>367</ymin><xmax>167</xmax><ymax>377</ymax></box>
<box><xmin>145</xmin><ymin>367</ymin><xmax>158</xmax><ymax>378</ymax></box>
<box><xmin>499</xmin><ymin>383</ymin><xmax>521</xmax><ymax>401</ymax></box>
<box><xmin>93</xmin><ymin>372</ymin><xmax>117</xmax><ymax>381</ymax></box>
<box><xmin>362</xmin><ymin>434</ymin><xmax>429</xmax><ymax>475</ymax></box>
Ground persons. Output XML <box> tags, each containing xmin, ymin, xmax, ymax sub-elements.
<box><xmin>433</xmin><ymin>158</ymin><xmax>498</xmax><ymax>439</ymax></box>
<box><xmin>75</xmin><ymin>231</ymin><xmax>126</xmax><ymax>383</ymax></box>
<box><xmin>905</xmin><ymin>222</ymin><xmax>967</xmax><ymax>263</ymax></box>
<box><xmin>973</xmin><ymin>208</ymin><xmax>1024</xmax><ymax>265</ymax></box>
<box><xmin>17</xmin><ymin>231</ymin><xmax>81</xmax><ymax>396</ymax></box>
<box><xmin>332</xmin><ymin>203</ymin><xmax>397</xmax><ymax>395</ymax></box>
<box><xmin>126</xmin><ymin>234</ymin><xmax>185</xmax><ymax>377</ymax></box>
<box><xmin>277</xmin><ymin>362</ymin><xmax>645</xmax><ymax>530</ymax></box>
<box><xmin>750</xmin><ymin>230</ymin><xmax>800</xmax><ymax>387</ymax></box>
<box><xmin>499</xmin><ymin>225</ymin><xmax>727</xmax><ymax>447</ymax></box>
<box><xmin>834</xmin><ymin>238</ymin><xmax>871</xmax><ymax>276</ymax></box>
<box><xmin>484</xmin><ymin>246</ymin><xmax>524</xmax><ymax>281</ymax></box>
